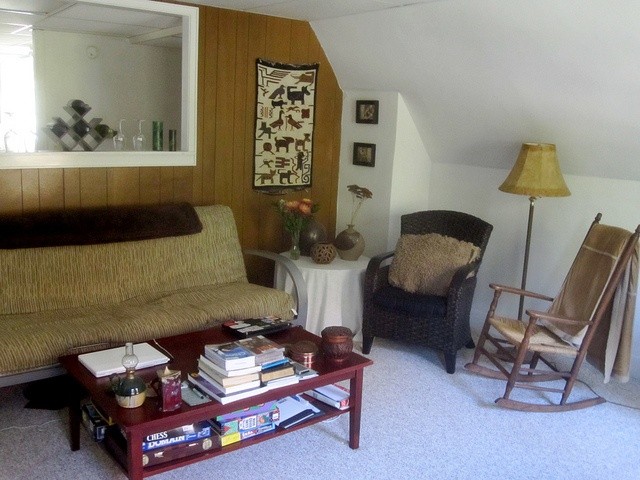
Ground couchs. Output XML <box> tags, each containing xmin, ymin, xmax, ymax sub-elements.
<box><xmin>0</xmin><ymin>204</ymin><xmax>313</xmax><ymax>386</ymax></box>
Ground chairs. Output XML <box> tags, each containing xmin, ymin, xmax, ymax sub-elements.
<box><xmin>362</xmin><ymin>210</ymin><xmax>493</xmax><ymax>375</ymax></box>
<box><xmin>464</xmin><ymin>212</ymin><xmax>640</xmax><ymax>413</ymax></box>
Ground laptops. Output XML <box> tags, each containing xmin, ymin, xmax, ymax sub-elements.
<box><xmin>77</xmin><ymin>342</ymin><xmax>171</xmax><ymax>378</ymax></box>
<box><xmin>222</xmin><ymin>314</ymin><xmax>293</xmax><ymax>341</ymax></box>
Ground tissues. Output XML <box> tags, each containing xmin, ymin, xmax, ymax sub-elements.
<box><xmin>156</xmin><ymin>366</ymin><xmax>182</xmax><ymax>414</ymax></box>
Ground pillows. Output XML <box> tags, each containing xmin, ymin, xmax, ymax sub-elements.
<box><xmin>389</xmin><ymin>231</ymin><xmax>476</xmax><ymax>295</ymax></box>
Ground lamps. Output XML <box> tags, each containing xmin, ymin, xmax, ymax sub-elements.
<box><xmin>498</xmin><ymin>141</ymin><xmax>572</xmax><ymax>320</ymax></box>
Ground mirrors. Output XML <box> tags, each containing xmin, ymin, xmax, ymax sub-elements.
<box><xmin>0</xmin><ymin>0</ymin><xmax>198</xmax><ymax>168</ymax></box>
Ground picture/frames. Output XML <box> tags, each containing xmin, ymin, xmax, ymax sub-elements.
<box><xmin>352</xmin><ymin>142</ymin><xmax>376</xmax><ymax>167</ymax></box>
<box><xmin>355</xmin><ymin>99</ymin><xmax>379</xmax><ymax>124</ymax></box>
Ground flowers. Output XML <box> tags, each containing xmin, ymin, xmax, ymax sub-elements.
<box><xmin>347</xmin><ymin>183</ymin><xmax>373</xmax><ymax>223</ymax></box>
<box><xmin>276</xmin><ymin>196</ymin><xmax>318</xmax><ymax>240</ymax></box>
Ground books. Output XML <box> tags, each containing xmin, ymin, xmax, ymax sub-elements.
<box><xmin>268</xmin><ymin>395</ymin><xmax>325</xmax><ymax>430</ymax></box>
<box><xmin>187</xmin><ymin>335</ymin><xmax>318</xmax><ymax>405</ymax></box>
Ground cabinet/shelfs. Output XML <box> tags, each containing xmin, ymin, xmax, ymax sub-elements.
<box><xmin>46</xmin><ymin>99</ymin><xmax>117</xmax><ymax>154</ymax></box>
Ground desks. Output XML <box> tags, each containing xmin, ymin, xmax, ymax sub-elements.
<box><xmin>274</xmin><ymin>251</ymin><xmax>372</xmax><ymax>340</ymax></box>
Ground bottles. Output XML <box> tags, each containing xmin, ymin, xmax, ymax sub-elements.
<box><xmin>170</xmin><ymin>130</ymin><xmax>177</xmax><ymax>151</ymax></box>
<box><xmin>152</xmin><ymin>121</ymin><xmax>163</xmax><ymax>152</ymax></box>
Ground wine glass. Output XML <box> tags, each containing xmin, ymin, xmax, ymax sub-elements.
<box><xmin>133</xmin><ymin>118</ymin><xmax>147</xmax><ymax>151</ymax></box>
<box><xmin>112</xmin><ymin>118</ymin><xmax>128</xmax><ymax>152</ymax></box>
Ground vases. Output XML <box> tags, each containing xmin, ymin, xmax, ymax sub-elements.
<box><xmin>335</xmin><ymin>224</ymin><xmax>365</xmax><ymax>262</ymax></box>
<box><xmin>290</xmin><ymin>237</ymin><xmax>301</xmax><ymax>259</ymax></box>
